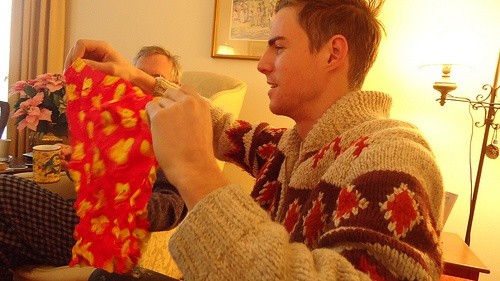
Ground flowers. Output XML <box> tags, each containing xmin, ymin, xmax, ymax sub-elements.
<box><xmin>10</xmin><ymin>73</ymin><xmax>69</xmax><ymax>137</ymax></box>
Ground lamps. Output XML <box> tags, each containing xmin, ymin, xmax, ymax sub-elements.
<box><xmin>423</xmin><ymin>34</ymin><xmax>500</xmax><ymax>247</ymax></box>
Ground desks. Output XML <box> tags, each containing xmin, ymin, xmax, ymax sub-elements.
<box><xmin>437</xmin><ymin>231</ymin><xmax>490</xmax><ymax>281</ymax></box>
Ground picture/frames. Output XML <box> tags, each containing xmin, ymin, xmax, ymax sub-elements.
<box><xmin>210</xmin><ymin>0</ymin><xmax>278</xmax><ymax>61</ymax></box>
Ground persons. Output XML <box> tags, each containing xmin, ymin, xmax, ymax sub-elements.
<box><xmin>61</xmin><ymin>46</ymin><xmax>190</xmax><ymax>233</ymax></box>
<box><xmin>13</xmin><ymin>0</ymin><xmax>446</xmax><ymax>281</ymax></box>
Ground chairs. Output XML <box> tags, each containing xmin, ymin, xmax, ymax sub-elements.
<box><xmin>0</xmin><ymin>101</ymin><xmax>12</xmax><ymax>139</ymax></box>
<box><xmin>136</xmin><ymin>70</ymin><xmax>249</xmax><ymax>281</ymax></box>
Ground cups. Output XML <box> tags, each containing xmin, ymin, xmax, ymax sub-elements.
<box><xmin>33</xmin><ymin>145</ymin><xmax>67</xmax><ymax>183</ymax></box>
<box><xmin>0</xmin><ymin>139</ymin><xmax>11</xmax><ymax>161</ymax></box>
